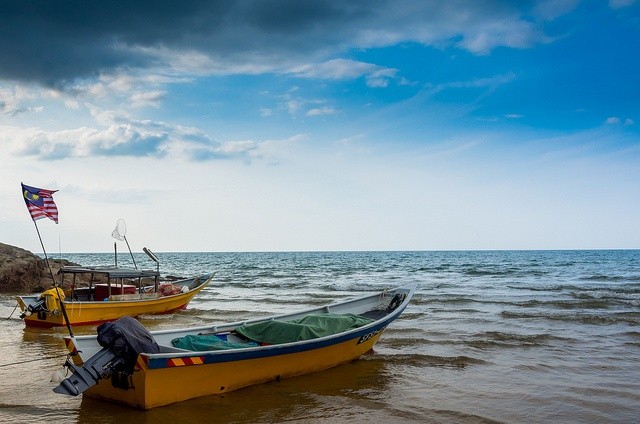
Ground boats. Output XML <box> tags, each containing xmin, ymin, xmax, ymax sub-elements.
<box><xmin>15</xmin><ymin>271</ymin><xmax>216</xmax><ymax>329</ymax></box>
<box><xmin>63</xmin><ymin>283</ymin><xmax>419</xmax><ymax>411</ymax></box>
